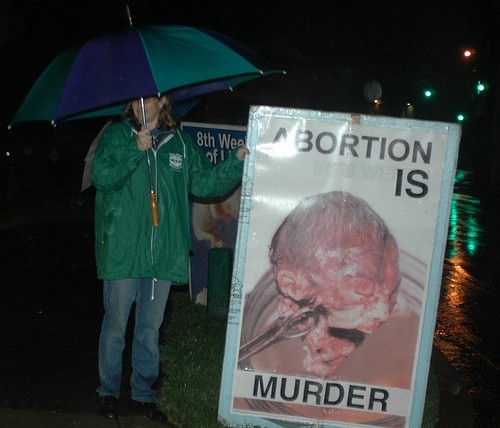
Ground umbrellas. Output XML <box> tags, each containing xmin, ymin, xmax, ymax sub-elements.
<box><xmin>6</xmin><ymin>5</ymin><xmax>286</xmax><ymax>227</ymax></box>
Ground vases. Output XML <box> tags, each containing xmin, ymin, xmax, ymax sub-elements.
<box><xmin>216</xmin><ymin>104</ymin><xmax>463</xmax><ymax>428</ymax></box>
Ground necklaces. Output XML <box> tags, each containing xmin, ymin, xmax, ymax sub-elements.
<box><xmin>89</xmin><ymin>76</ymin><xmax>250</xmax><ymax>422</ymax></box>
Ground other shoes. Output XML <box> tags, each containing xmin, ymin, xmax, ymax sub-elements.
<box><xmin>100</xmin><ymin>395</ymin><xmax>118</xmax><ymax>419</ymax></box>
<box><xmin>131</xmin><ymin>400</ymin><xmax>167</xmax><ymax>422</ymax></box>
